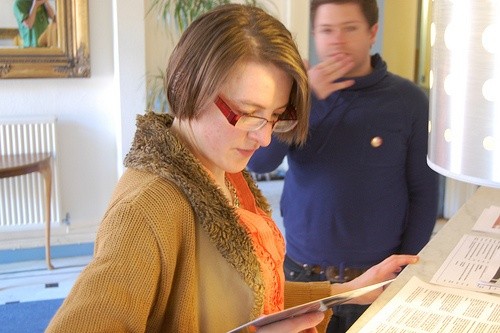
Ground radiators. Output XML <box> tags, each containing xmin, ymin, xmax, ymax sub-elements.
<box><xmin>1</xmin><ymin>117</ymin><xmax>61</xmax><ymax>232</ymax></box>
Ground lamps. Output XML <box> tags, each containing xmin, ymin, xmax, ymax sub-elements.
<box><xmin>427</xmin><ymin>0</ymin><xmax>500</xmax><ymax>189</ymax></box>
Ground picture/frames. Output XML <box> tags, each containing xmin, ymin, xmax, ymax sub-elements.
<box><xmin>0</xmin><ymin>0</ymin><xmax>91</xmax><ymax>78</ymax></box>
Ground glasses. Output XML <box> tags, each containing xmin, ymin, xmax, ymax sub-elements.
<box><xmin>211</xmin><ymin>94</ymin><xmax>300</xmax><ymax>133</ymax></box>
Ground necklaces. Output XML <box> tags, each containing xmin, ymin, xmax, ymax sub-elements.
<box><xmin>225</xmin><ymin>175</ymin><xmax>239</xmax><ymax>208</ymax></box>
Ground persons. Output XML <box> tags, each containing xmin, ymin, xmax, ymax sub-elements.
<box><xmin>244</xmin><ymin>0</ymin><xmax>438</xmax><ymax>333</ymax></box>
<box><xmin>13</xmin><ymin>0</ymin><xmax>58</xmax><ymax>48</ymax></box>
<box><xmin>43</xmin><ymin>0</ymin><xmax>419</xmax><ymax>333</ymax></box>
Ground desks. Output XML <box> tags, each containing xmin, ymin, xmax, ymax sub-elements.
<box><xmin>0</xmin><ymin>153</ymin><xmax>54</xmax><ymax>270</ymax></box>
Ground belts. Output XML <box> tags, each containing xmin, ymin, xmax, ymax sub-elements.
<box><xmin>303</xmin><ymin>262</ymin><xmax>373</xmax><ymax>285</ymax></box>
